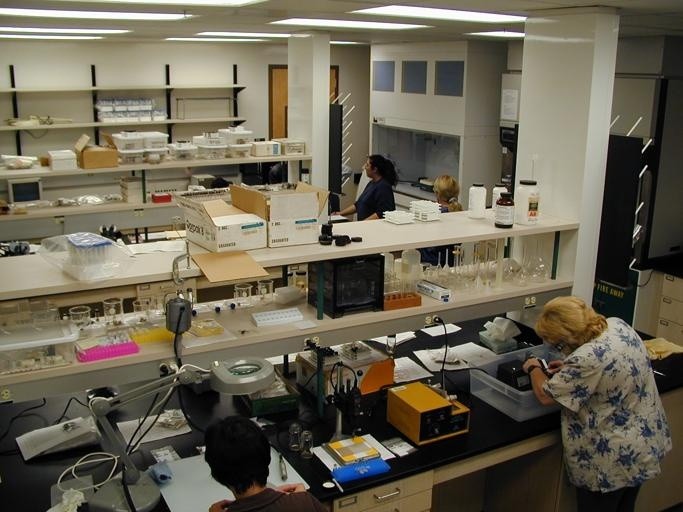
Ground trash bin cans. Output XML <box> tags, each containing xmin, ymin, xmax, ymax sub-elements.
<box><xmin>341</xmin><ymin>164</ymin><xmax>354</xmax><ymax>221</ymax></box>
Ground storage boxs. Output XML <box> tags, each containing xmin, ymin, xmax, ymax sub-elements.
<box><xmin>46</xmin><ymin>132</ymin><xmax>120</xmax><ymax>171</ymax></box>
<box><xmin>171</xmin><ymin>181</ymin><xmax>332</xmax><ymax>256</ymax></box>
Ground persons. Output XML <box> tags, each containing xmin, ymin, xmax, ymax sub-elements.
<box><xmin>417</xmin><ymin>175</ymin><xmax>464</xmax><ymax>267</ymax></box>
<box><xmin>330</xmin><ymin>154</ymin><xmax>399</xmax><ymax>221</ymax></box>
<box><xmin>521</xmin><ymin>295</ymin><xmax>674</xmax><ymax>512</ymax></box>
<box><xmin>204</xmin><ymin>415</ymin><xmax>332</xmax><ymax>512</ymax></box>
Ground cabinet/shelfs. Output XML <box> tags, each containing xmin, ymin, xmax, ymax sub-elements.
<box><xmin>633</xmin><ymin>268</ymin><xmax>683</xmax><ymax>349</ymax></box>
<box><xmin>0</xmin><ymin>203</ymin><xmax>579</xmax><ymax>395</ymax></box>
<box><xmin>3</xmin><ymin>84</ymin><xmax>249</xmax><ymax>158</ymax></box>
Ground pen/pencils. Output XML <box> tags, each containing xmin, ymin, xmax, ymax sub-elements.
<box><xmin>279</xmin><ymin>454</ymin><xmax>287</xmax><ymax>481</ymax></box>
<box><xmin>333</xmin><ymin>478</ymin><xmax>343</xmax><ymax>493</ymax></box>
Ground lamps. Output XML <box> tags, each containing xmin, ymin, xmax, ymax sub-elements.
<box><xmin>84</xmin><ymin>352</ymin><xmax>274</xmax><ymax>510</ymax></box>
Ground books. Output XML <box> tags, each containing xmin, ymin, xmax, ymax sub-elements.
<box><xmin>328</xmin><ymin>436</ymin><xmax>380</xmax><ymax>465</ymax></box>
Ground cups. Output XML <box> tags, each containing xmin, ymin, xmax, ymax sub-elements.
<box><xmin>68</xmin><ymin>295</ymin><xmax>153</xmax><ymax>332</ymax></box>
<box><xmin>233</xmin><ymin>280</ymin><xmax>274</xmax><ymax>310</ymax></box>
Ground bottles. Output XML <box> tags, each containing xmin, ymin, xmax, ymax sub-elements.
<box><xmin>294</xmin><ymin>270</ymin><xmax>306</xmax><ymax>304</ymax></box>
<box><xmin>288</xmin><ymin>423</ymin><xmax>312</xmax><ymax>459</ymax></box>
<box><xmin>380</xmin><ymin>240</ymin><xmax>550</xmax><ymax>301</ymax></box>
<box><xmin>469</xmin><ymin>178</ymin><xmax>541</xmax><ymax>227</ymax></box>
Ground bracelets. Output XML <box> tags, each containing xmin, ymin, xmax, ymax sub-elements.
<box><xmin>528</xmin><ymin>364</ymin><xmax>543</xmax><ymax>373</ymax></box>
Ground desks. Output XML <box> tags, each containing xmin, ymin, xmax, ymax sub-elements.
<box><xmin>0</xmin><ymin>310</ymin><xmax>683</xmax><ymax>512</ymax></box>
